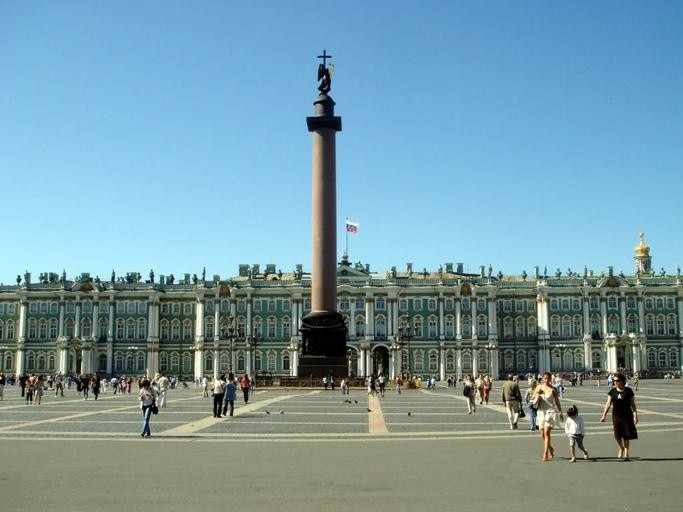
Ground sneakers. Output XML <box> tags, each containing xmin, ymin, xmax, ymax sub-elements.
<box><xmin>584</xmin><ymin>449</ymin><xmax>589</xmax><ymax>460</ymax></box>
<box><xmin>569</xmin><ymin>457</ymin><xmax>576</xmax><ymax>463</ymax></box>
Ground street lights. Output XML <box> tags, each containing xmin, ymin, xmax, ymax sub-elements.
<box><xmin>188</xmin><ymin>345</ymin><xmax>199</xmax><ymax>380</ymax></box>
<box><xmin>388</xmin><ymin>336</ymin><xmax>401</xmax><ymax>379</ymax></box>
<box><xmin>128</xmin><ymin>346</ymin><xmax>139</xmax><ymax>378</ymax></box>
<box><xmin>287</xmin><ymin>344</ymin><xmax>297</xmax><ymax>376</ymax></box>
<box><xmin>397</xmin><ymin>313</ymin><xmax>421</xmax><ymax>374</ymax></box>
<box><xmin>219</xmin><ymin>314</ymin><xmax>240</xmax><ymax>376</ymax></box>
<box><xmin>485</xmin><ymin>343</ymin><xmax>497</xmax><ymax>376</ymax></box>
<box><xmin>245</xmin><ymin>324</ymin><xmax>263</xmax><ymax>374</ymax></box>
<box><xmin>360</xmin><ymin>342</ymin><xmax>370</xmax><ymax>377</ymax></box>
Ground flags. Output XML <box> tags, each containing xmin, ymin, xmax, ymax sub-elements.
<box><xmin>346</xmin><ymin>222</ymin><xmax>358</xmax><ymax>233</ymax></box>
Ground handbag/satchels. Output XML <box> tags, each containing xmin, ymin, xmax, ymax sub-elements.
<box><xmin>533</xmin><ymin>393</ymin><xmax>541</xmax><ymax>409</ymax></box>
<box><xmin>151</xmin><ymin>399</ymin><xmax>158</xmax><ymax>414</ymax></box>
<box><xmin>463</xmin><ymin>386</ymin><xmax>471</xmax><ymax>396</ymax></box>
<box><xmin>517</xmin><ymin>408</ymin><xmax>525</xmax><ymax>418</ymax></box>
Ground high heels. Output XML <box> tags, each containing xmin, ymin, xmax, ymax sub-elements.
<box><xmin>543</xmin><ymin>447</ymin><xmax>554</xmax><ymax>459</ymax></box>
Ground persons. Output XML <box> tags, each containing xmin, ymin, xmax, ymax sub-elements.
<box><xmin>501</xmin><ymin>373</ymin><xmax>523</xmax><ymax>429</ymax></box>
<box><xmin>138</xmin><ymin>379</ymin><xmax>154</xmax><ymax>437</ymax></box>
<box><xmin>0</xmin><ymin>371</ymin><xmax>254</xmax><ymax>418</ymax></box>
<box><xmin>513</xmin><ymin>372</ymin><xmax>583</xmax><ymax>396</ymax></box>
<box><xmin>564</xmin><ymin>405</ymin><xmax>588</xmax><ymax>463</ymax></box>
<box><xmin>523</xmin><ymin>378</ymin><xmax>540</xmax><ymax>429</ymax></box>
<box><xmin>324</xmin><ymin>372</ymin><xmax>401</xmax><ymax>397</ymax></box>
<box><xmin>531</xmin><ymin>372</ymin><xmax>563</xmax><ymax>461</ymax></box>
<box><xmin>599</xmin><ymin>372</ymin><xmax>638</xmax><ymax>461</ymax></box>
<box><xmin>606</xmin><ymin>370</ymin><xmax>631</xmax><ymax>387</ymax></box>
<box><xmin>462</xmin><ymin>373</ymin><xmax>495</xmax><ymax>415</ymax></box>
<box><xmin>426</xmin><ymin>374</ymin><xmax>458</xmax><ymax>391</ymax></box>
